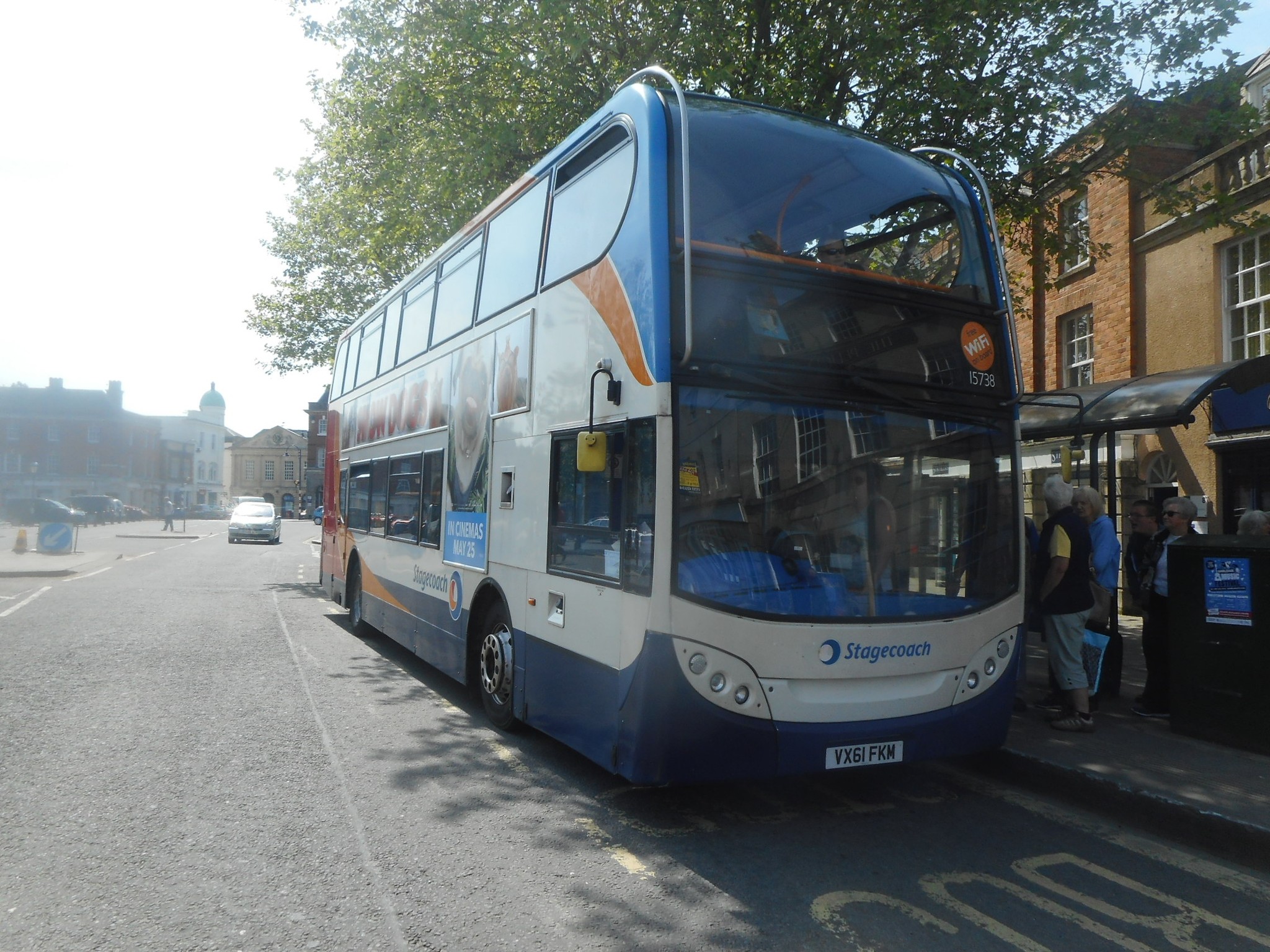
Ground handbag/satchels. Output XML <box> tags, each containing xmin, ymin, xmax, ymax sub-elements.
<box><xmin>1063</xmin><ymin>629</ymin><xmax>1110</xmax><ymax>697</ymax></box>
<box><xmin>1085</xmin><ymin>580</ymin><xmax>1111</xmax><ymax>623</ymax></box>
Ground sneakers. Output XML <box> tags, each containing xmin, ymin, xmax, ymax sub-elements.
<box><xmin>1051</xmin><ymin>713</ymin><xmax>1096</xmax><ymax>732</ymax></box>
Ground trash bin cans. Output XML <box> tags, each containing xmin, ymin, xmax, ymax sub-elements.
<box><xmin>1165</xmin><ymin>534</ymin><xmax>1270</xmax><ymax>755</ymax></box>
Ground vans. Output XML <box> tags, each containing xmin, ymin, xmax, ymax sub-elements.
<box><xmin>231</xmin><ymin>496</ymin><xmax>266</xmax><ymax>507</ymax></box>
<box><xmin>61</xmin><ymin>495</ymin><xmax>114</xmax><ymax>524</ymax></box>
<box><xmin>5</xmin><ymin>498</ymin><xmax>86</xmax><ymax>527</ymax></box>
<box><xmin>228</xmin><ymin>501</ymin><xmax>282</xmax><ymax>545</ymax></box>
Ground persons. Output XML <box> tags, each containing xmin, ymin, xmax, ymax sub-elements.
<box><xmin>1007</xmin><ymin>517</ymin><xmax>1037</xmax><ymax>711</ymax></box>
<box><xmin>1236</xmin><ymin>509</ymin><xmax>1270</xmax><ymax>535</ymax></box>
<box><xmin>1033</xmin><ymin>472</ymin><xmax>1094</xmax><ymax>731</ymax></box>
<box><xmin>845</xmin><ymin>464</ymin><xmax>897</xmax><ymax>591</ymax></box>
<box><xmin>1123</xmin><ymin>500</ymin><xmax>1163</xmax><ymax>703</ymax></box>
<box><xmin>1070</xmin><ymin>486</ymin><xmax>1122</xmax><ymax>634</ymax></box>
<box><xmin>816</xmin><ymin>236</ymin><xmax>865</xmax><ymax>271</ymax></box>
<box><xmin>161</xmin><ymin>497</ymin><xmax>174</xmax><ymax>532</ymax></box>
<box><xmin>1130</xmin><ymin>497</ymin><xmax>1199</xmax><ymax>716</ymax></box>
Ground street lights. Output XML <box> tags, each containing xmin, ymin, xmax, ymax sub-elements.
<box><xmin>282</xmin><ymin>444</ymin><xmax>301</xmax><ymax>520</ymax></box>
<box><xmin>30</xmin><ymin>462</ymin><xmax>39</xmax><ymax>498</ymax></box>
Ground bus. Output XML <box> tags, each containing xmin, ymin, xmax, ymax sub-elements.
<box><xmin>318</xmin><ymin>64</ymin><xmax>1084</xmax><ymax>791</ymax></box>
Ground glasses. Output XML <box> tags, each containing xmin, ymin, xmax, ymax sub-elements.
<box><xmin>849</xmin><ymin>476</ymin><xmax>868</xmax><ymax>486</ymax></box>
<box><xmin>1160</xmin><ymin>511</ymin><xmax>1182</xmax><ymax>517</ymax></box>
<box><xmin>1127</xmin><ymin>513</ymin><xmax>1153</xmax><ymax>520</ymax></box>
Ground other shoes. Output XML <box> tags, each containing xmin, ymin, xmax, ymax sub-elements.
<box><xmin>1013</xmin><ymin>695</ymin><xmax>1027</xmax><ymax>711</ymax></box>
<box><xmin>1035</xmin><ymin>699</ymin><xmax>1062</xmax><ymax>709</ymax></box>
<box><xmin>1130</xmin><ymin>706</ymin><xmax>1171</xmax><ymax>718</ymax></box>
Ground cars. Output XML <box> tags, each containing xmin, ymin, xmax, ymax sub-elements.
<box><xmin>114</xmin><ymin>499</ymin><xmax>231</xmax><ymax>522</ymax></box>
<box><xmin>313</xmin><ymin>506</ymin><xmax>323</xmax><ymax>525</ymax></box>
<box><xmin>340</xmin><ymin>503</ymin><xmax>440</xmax><ymax>544</ymax></box>
